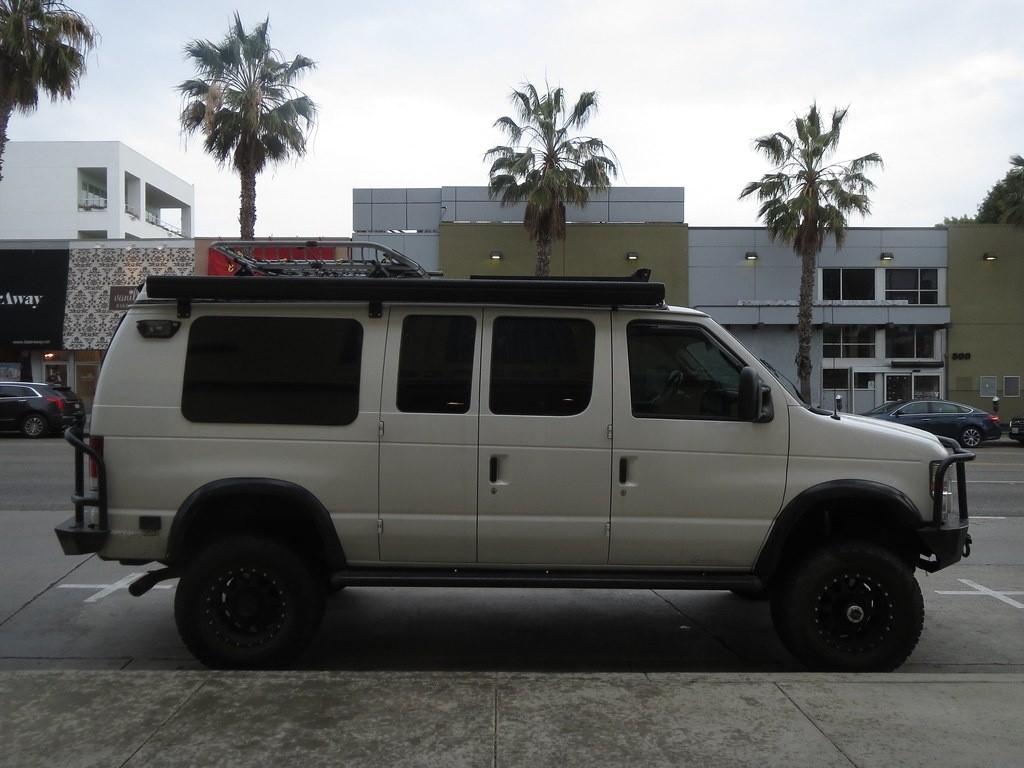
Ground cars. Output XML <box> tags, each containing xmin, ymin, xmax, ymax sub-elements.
<box><xmin>860</xmin><ymin>396</ymin><xmax>1001</xmax><ymax>446</ymax></box>
<box><xmin>0</xmin><ymin>379</ymin><xmax>88</xmax><ymax>439</ymax></box>
<box><xmin>1007</xmin><ymin>414</ymin><xmax>1024</xmax><ymax>444</ymax></box>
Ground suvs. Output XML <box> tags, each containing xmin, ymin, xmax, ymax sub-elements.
<box><xmin>57</xmin><ymin>238</ymin><xmax>977</xmax><ymax>676</ymax></box>
